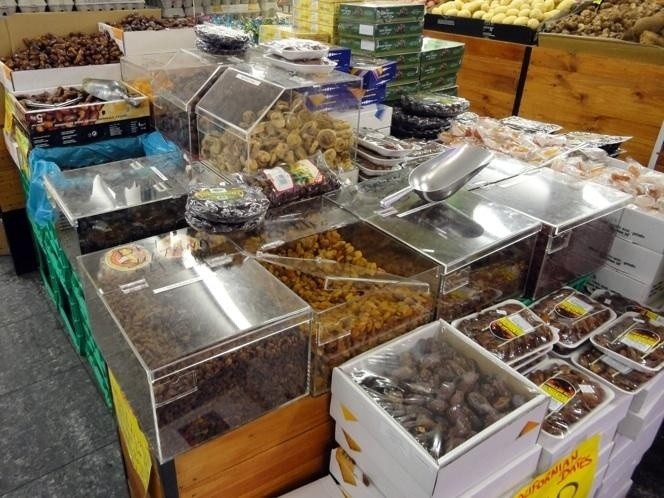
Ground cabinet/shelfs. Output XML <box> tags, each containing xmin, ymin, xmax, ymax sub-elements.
<box><xmin>424</xmin><ymin>0</ymin><xmax>586</xmax><ymax>118</ymax></box>
<box><xmin>0</xmin><ymin>84</ymin><xmax>38</xmax><ymax>276</ymax></box>
<box><xmin>27</xmin><ymin>188</ymin><xmax>335</xmax><ymax>498</ymax></box>
<box><xmin>518</xmin><ymin>0</ymin><xmax>664</xmax><ymax>173</ymax></box>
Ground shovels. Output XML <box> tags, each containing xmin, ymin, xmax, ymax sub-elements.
<box><xmin>380</xmin><ymin>143</ymin><xmax>496</xmax><ymax>208</ymax></box>
<box><xmin>83</xmin><ymin>78</ymin><xmax>141</xmax><ymax>107</ymax></box>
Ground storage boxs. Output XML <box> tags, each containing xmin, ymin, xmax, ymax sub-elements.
<box><xmin>590</xmin><ymin>199</ymin><xmax>664</xmax><ymax>307</ymax></box>
<box><xmin>28</xmin><ymin>131</ymin><xmax>172</xmax><ymax>221</ymax></box>
<box><xmin>5</xmin><ymin>93</ymin><xmax>151</xmax><ymax>173</ymax></box>
<box><xmin>543</xmin><ymin>374</ymin><xmax>663</xmax><ymax>498</ymax></box>
<box><xmin>324</xmin><ymin>167</ymin><xmax>541</xmax><ymax>324</ymax></box>
<box><xmin>98</xmin><ymin>22</ymin><xmax>196</xmax><ymax>56</ymax></box>
<box><xmin>42</xmin><ymin>151</ymin><xmax>213</xmax><ymax>280</ymax></box>
<box><xmin>74</xmin><ymin>226</ymin><xmax>313</xmax><ymax>466</ymax></box>
<box><xmin>0</xmin><ymin>60</ymin><xmax>122</xmax><ymax>91</ymax></box>
<box><xmin>151</xmin><ymin>49</ymin><xmax>265</xmax><ymax>160</ymax></box>
<box><xmin>330</xmin><ymin>448</ymin><xmax>384</xmax><ymax>498</ymax></box>
<box><xmin>118</xmin><ymin>50</ymin><xmax>179</xmax><ymax>124</ymax></box>
<box><xmin>228</xmin><ymin>195</ymin><xmax>442</xmax><ymax>397</ymax></box>
<box><xmin>328</xmin><ymin>103</ymin><xmax>393</xmax><ymax>135</ymax></box>
<box><xmin>196</xmin><ymin>64</ymin><xmax>363</xmax><ymax>181</ymax></box>
<box><xmin>0</xmin><ymin>8</ymin><xmax>161</xmax><ymax>60</ymax></box>
<box><xmin>259</xmin><ymin>0</ymin><xmax>465</xmax><ymax>110</ymax></box>
<box><xmin>462</xmin><ymin>156</ymin><xmax>635</xmax><ymax>303</ymax></box>
<box><xmin>335</xmin><ymin>422</ymin><xmax>542</xmax><ymax>498</ymax></box>
<box><xmin>329</xmin><ymin>319</ymin><xmax>551</xmax><ymax>498</ymax></box>
<box><xmin>274</xmin><ymin>473</ymin><xmax>346</xmax><ymax>497</ymax></box>
